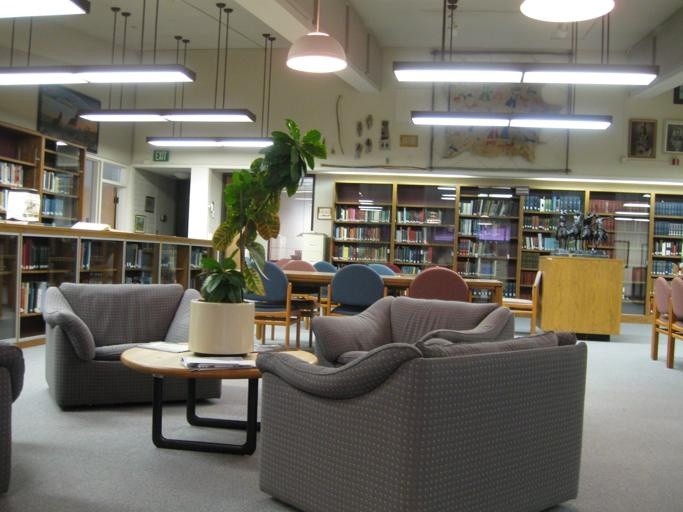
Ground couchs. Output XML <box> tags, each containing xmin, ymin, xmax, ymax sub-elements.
<box><xmin>310</xmin><ymin>293</ymin><xmax>515</xmax><ymax>358</ymax></box>
<box><xmin>253</xmin><ymin>330</ymin><xmax>586</xmax><ymax>512</ymax></box>
<box><xmin>40</xmin><ymin>283</ymin><xmax>223</xmax><ymax>407</ymax></box>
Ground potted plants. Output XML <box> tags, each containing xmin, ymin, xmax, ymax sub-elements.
<box><xmin>187</xmin><ymin>114</ymin><xmax>328</xmax><ymax>356</ymax></box>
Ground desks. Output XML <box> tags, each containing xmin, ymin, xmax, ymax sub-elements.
<box><xmin>119</xmin><ymin>340</ymin><xmax>319</xmax><ymax>454</ymax></box>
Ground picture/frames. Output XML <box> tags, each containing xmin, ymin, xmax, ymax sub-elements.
<box><xmin>672</xmin><ymin>87</ymin><xmax>682</xmax><ymax>105</ymax></box>
<box><xmin>627</xmin><ymin>118</ymin><xmax>657</xmax><ymax>159</ymax></box>
<box><xmin>36</xmin><ymin>81</ymin><xmax>101</xmax><ymax>155</ymax></box>
<box><xmin>663</xmin><ymin>120</ymin><xmax>683</xmax><ymax>155</ymax></box>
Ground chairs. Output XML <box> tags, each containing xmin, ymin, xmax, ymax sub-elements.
<box><xmin>241</xmin><ymin>258</ymin><xmax>507</xmax><ymax>337</ymax></box>
<box><xmin>650</xmin><ymin>275</ymin><xmax>671</xmax><ymax>366</ymax></box>
<box><xmin>669</xmin><ymin>275</ymin><xmax>683</xmax><ymax>371</ymax></box>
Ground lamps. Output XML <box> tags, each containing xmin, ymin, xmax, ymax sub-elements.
<box><xmin>144</xmin><ymin>33</ymin><xmax>283</xmax><ymax>149</ymax></box>
<box><xmin>79</xmin><ymin>1</ymin><xmax>257</xmax><ymax>124</ymax></box>
<box><xmin>388</xmin><ymin>1</ymin><xmax>659</xmax><ymax>86</ymax></box>
<box><xmin>285</xmin><ymin>0</ymin><xmax>347</xmax><ymax>74</ymax></box>
<box><xmin>409</xmin><ymin>4</ymin><xmax>615</xmax><ymax>131</ymax></box>
<box><xmin>0</xmin><ymin>1</ymin><xmax>200</xmax><ymax>87</ymax></box>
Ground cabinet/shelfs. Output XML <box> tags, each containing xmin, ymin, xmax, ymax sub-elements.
<box><xmin>3</xmin><ymin>128</ymin><xmax>82</xmax><ymax>232</ymax></box>
<box><xmin>518</xmin><ymin>187</ymin><xmax>589</xmax><ymax>299</ymax></box>
<box><xmin>1</xmin><ymin>232</ymin><xmax>217</xmax><ymax>353</ymax></box>
<box><xmin>587</xmin><ymin>194</ymin><xmax>654</xmax><ymax>317</ymax></box>
<box><xmin>391</xmin><ymin>182</ymin><xmax>460</xmax><ymax>272</ymax></box>
<box><xmin>334</xmin><ymin>181</ymin><xmax>393</xmax><ymax>268</ymax></box>
<box><xmin>457</xmin><ymin>188</ymin><xmax>523</xmax><ymax>298</ymax></box>
<box><xmin>647</xmin><ymin>193</ymin><xmax>683</xmax><ymax>315</ymax></box>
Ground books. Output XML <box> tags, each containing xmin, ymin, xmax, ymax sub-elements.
<box><xmin>335</xmin><ymin>203</ymin><xmax>391</xmax><ymax>271</ymax></box>
<box><xmin>652</xmin><ymin>195</ymin><xmax>683</xmax><ymax>275</ymax></box>
<box><xmin>520</xmin><ymin>195</ymin><xmax>584</xmax><ymax>301</ymax></box>
<box><xmin>472</xmin><ymin>288</ymin><xmax>492</xmax><ymax>303</ymax></box>
<box><xmin>396</xmin><ymin>286</ymin><xmax>409</xmax><ymax>296</ymax></box>
<box><xmin>589</xmin><ymin>195</ymin><xmax>650</xmax><ymax>302</ymax></box>
<box><xmin>394</xmin><ymin>208</ymin><xmax>454</xmax><ymax>274</ymax></box>
<box><xmin>0</xmin><ymin>160</ymin><xmax>208</xmax><ymax>316</ymax></box>
<box><xmin>457</xmin><ymin>199</ymin><xmax>519</xmax><ymax>299</ymax></box>
